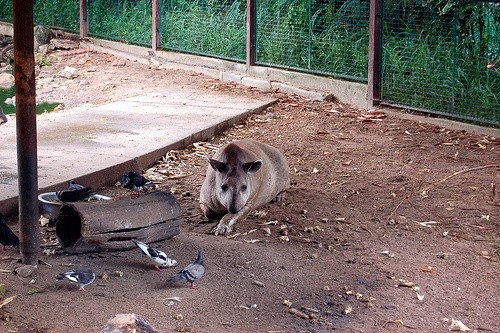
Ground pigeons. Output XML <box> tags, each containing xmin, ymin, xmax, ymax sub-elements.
<box><xmin>120</xmin><ymin>171</ymin><xmax>151</xmax><ymax>198</ymax></box>
<box><xmin>55</xmin><ymin>186</ymin><xmax>95</xmax><ymax>203</ymax></box>
<box><xmin>167</xmin><ymin>247</ymin><xmax>206</xmax><ymax>289</ymax></box>
<box><xmin>55</xmin><ymin>269</ymin><xmax>96</xmax><ymax>292</ymax></box>
<box><xmin>130</xmin><ymin>240</ymin><xmax>179</xmax><ymax>272</ymax></box>
<box><xmin>69</xmin><ymin>180</ymin><xmax>87</xmax><ymax>191</ymax></box>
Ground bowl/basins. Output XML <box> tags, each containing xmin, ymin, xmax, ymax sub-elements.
<box><xmin>38</xmin><ymin>192</ymin><xmax>112</xmax><ymax>218</ymax></box>
